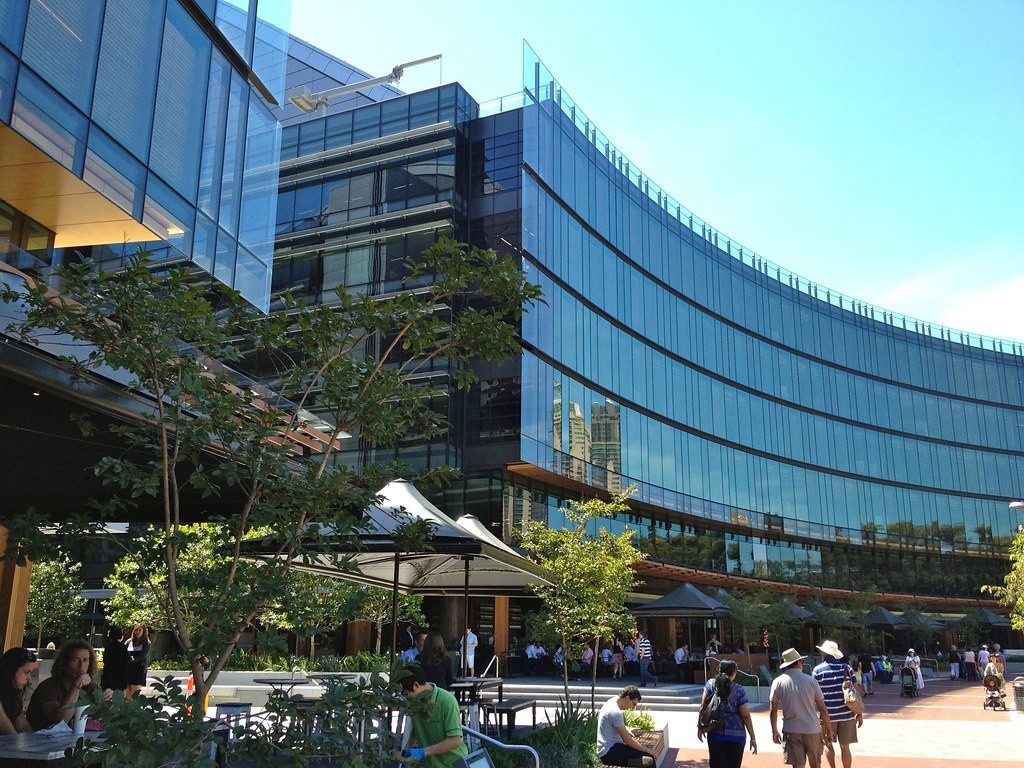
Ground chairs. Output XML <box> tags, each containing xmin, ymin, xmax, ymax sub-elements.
<box><xmin>552</xmin><ymin>654</ymin><xmax>687</xmax><ymax>683</ymax></box>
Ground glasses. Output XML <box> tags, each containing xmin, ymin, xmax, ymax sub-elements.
<box><xmin>21</xmin><ymin>651</ymin><xmax>33</xmax><ymax>660</ymax></box>
<box><xmin>796</xmin><ymin>660</ymin><xmax>804</xmax><ymax>664</ymax></box>
<box><xmin>632</xmin><ymin>699</ymin><xmax>636</xmax><ymax>707</ymax></box>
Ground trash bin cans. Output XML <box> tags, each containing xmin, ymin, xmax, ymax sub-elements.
<box><xmin>1012</xmin><ymin>676</ymin><xmax>1024</xmax><ymax>711</ymax></box>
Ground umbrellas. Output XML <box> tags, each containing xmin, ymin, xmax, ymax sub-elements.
<box><xmin>217</xmin><ymin>478</ymin><xmax>558</xmax><ymax>733</ymax></box>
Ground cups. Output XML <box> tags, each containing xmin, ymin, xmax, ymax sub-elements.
<box><xmin>74</xmin><ymin>705</ymin><xmax>90</xmax><ymax>734</ymax></box>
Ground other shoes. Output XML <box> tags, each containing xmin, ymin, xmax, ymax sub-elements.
<box><xmin>869</xmin><ymin>692</ymin><xmax>874</xmax><ymax>697</ymax></box>
<box><xmin>627</xmin><ymin>756</ymin><xmax>653</xmax><ymax>767</ymax></box>
<box><xmin>619</xmin><ymin>676</ymin><xmax>623</xmax><ymax>680</ymax></box>
<box><xmin>655</xmin><ymin>676</ymin><xmax>658</xmax><ymax>687</ymax></box>
<box><xmin>863</xmin><ymin>692</ymin><xmax>868</xmax><ymax>698</ymax></box>
<box><xmin>613</xmin><ymin>675</ymin><xmax>616</xmax><ymax>680</ymax></box>
<box><xmin>638</xmin><ymin>684</ymin><xmax>646</xmax><ymax>687</ymax></box>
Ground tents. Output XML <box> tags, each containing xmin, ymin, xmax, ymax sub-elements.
<box><xmin>627</xmin><ymin>583</ymin><xmax>1013</xmax><ymax>631</ymax></box>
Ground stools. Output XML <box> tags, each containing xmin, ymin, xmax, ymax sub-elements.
<box><xmin>288</xmin><ymin>696</ymin><xmax>501</xmax><ymax>753</ymax></box>
<box><xmin>214</xmin><ymin>702</ymin><xmax>253</xmax><ymax>741</ymax></box>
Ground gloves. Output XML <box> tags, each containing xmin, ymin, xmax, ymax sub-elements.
<box><xmin>402</xmin><ymin>748</ymin><xmax>424</xmax><ymax>761</ymax></box>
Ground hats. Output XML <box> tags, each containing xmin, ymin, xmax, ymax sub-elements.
<box><xmin>908</xmin><ymin>648</ymin><xmax>915</xmax><ymax>653</ymax></box>
<box><xmin>983</xmin><ymin>645</ymin><xmax>988</xmax><ymax>649</ymax></box>
<box><xmin>779</xmin><ymin>648</ymin><xmax>809</xmax><ymax>669</ymax></box>
<box><xmin>950</xmin><ymin>645</ymin><xmax>957</xmax><ymax>651</ymax></box>
<box><xmin>816</xmin><ymin>639</ymin><xmax>844</xmax><ymax>660</ymax></box>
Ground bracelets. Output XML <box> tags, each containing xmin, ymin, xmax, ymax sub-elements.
<box><xmin>73</xmin><ymin>684</ymin><xmax>80</xmax><ymax>689</ymax></box>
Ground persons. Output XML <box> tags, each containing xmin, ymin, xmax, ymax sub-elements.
<box><xmin>949</xmin><ymin>644</ymin><xmax>1006</xmax><ymax>691</ymax></box>
<box><xmin>602</xmin><ymin>635</ymin><xmax>634</xmax><ymax>680</ymax></box>
<box><xmin>579</xmin><ymin>641</ymin><xmax>593</xmax><ymax>669</ymax></box>
<box><xmin>414</xmin><ymin>631</ymin><xmax>453</xmax><ymax>690</ymax></box>
<box><xmin>811</xmin><ymin>640</ymin><xmax>863</xmax><ymax>768</ymax></box>
<box><xmin>732</xmin><ymin>643</ymin><xmax>744</xmax><ymax>654</ymax></box>
<box><xmin>706</xmin><ymin>634</ymin><xmax>721</xmax><ymax>656</ymax></box>
<box><xmin>853</xmin><ymin>646</ymin><xmax>894</xmax><ymax>699</ymax></box>
<box><xmin>597</xmin><ymin>685</ymin><xmax>658</xmax><ymax>768</ymax></box>
<box><xmin>26</xmin><ymin>640</ymin><xmax>114</xmax><ymax>731</ymax></box>
<box><xmin>525</xmin><ymin>640</ymin><xmax>546</xmax><ymax>660</ymax></box>
<box><xmin>403</xmin><ymin>624</ymin><xmax>416</xmax><ymax>650</ymax></box>
<box><xmin>675</xmin><ymin>643</ymin><xmax>690</xmax><ymax>679</ymax></box>
<box><xmin>394</xmin><ymin>666</ymin><xmax>469</xmax><ymax>768</ymax></box>
<box><xmin>904</xmin><ymin>647</ymin><xmax>924</xmax><ymax>689</ymax></box>
<box><xmin>638</xmin><ymin>631</ymin><xmax>658</xmax><ymax>687</ymax></box>
<box><xmin>0</xmin><ymin>647</ymin><xmax>37</xmax><ymax>735</ymax></box>
<box><xmin>101</xmin><ymin>622</ymin><xmax>152</xmax><ymax>700</ymax></box>
<box><xmin>769</xmin><ymin>648</ymin><xmax>833</xmax><ymax>768</ymax></box>
<box><xmin>459</xmin><ymin>625</ymin><xmax>478</xmax><ymax>676</ymax></box>
<box><xmin>401</xmin><ymin>629</ymin><xmax>428</xmax><ymax>664</ymax></box>
<box><xmin>697</xmin><ymin>659</ymin><xmax>757</xmax><ymax>768</ymax></box>
<box><xmin>553</xmin><ymin>646</ymin><xmax>567</xmax><ymax>669</ymax></box>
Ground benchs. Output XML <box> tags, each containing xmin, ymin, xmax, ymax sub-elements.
<box><xmin>693</xmin><ymin>654</ymin><xmax>769</xmax><ymax>685</ymax></box>
<box><xmin>598</xmin><ymin>730</ymin><xmax>665</xmax><ymax>768</ymax></box>
<box><xmin>484</xmin><ymin>698</ymin><xmax>536</xmax><ymax>742</ymax></box>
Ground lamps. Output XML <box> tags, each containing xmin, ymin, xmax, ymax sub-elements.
<box><xmin>33</xmin><ymin>388</ymin><xmax>40</xmax><ymax>397</ymax></box>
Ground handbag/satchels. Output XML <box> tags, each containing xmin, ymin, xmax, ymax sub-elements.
<box><xmin>842</xmin><ymin>663</ymin><xmax>864</xmax><ymax>715</ymax></box>
<box><xmin>699</xmin><ymin>677</ymin><xmax>722</xmax><ymax>733</ymax></box>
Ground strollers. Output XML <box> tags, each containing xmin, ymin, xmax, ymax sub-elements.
<box><xmin>983</xmin><ymin>675</ymin><xmax>1008</xmax><ymax>711</ymax></box>
<box><xmin>899</xmin><ymin>666</ymin><xmax>922</xmax><ymax>698</ymax></box>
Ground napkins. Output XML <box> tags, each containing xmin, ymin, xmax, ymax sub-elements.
<box><xmin>35</xmin><ymin>720</ymin><xmax>73</xmax><ymax>734</ymax></box>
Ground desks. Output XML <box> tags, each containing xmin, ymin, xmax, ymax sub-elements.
<box><xmin>0</xmin><ymin>725</ymin><xmax>230</xmax><ymax>768</ymax></box>
<box><xmin>252</xmin><ymin>679</ymin><xmax>309</xmax><ymax>756</ymax></box>
<box><xmin>305</xmin><ymin>675</ymin><xmax>357</xmax><ymax>694</ymax></box>
<box><xmin>448</xmin><ymin>680</ymin><xmax>504</xmax><ymax>731</ymax></box>
